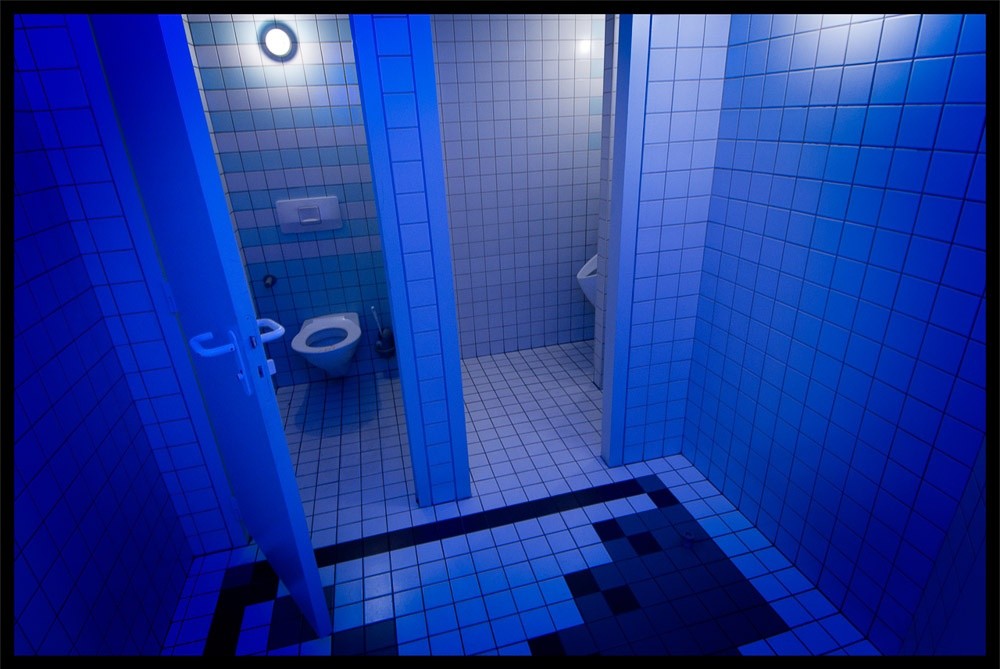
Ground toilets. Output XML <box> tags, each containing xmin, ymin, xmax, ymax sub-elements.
<box><xmin>291</xmin><ymin>310</ymin><xmax>362</xmax><ymax>377</ymax></box>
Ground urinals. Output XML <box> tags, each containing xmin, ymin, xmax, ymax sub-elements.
<box><xmin>577</xmin><ymin>254</ymin><xmax>598</xmax><ymax>304</ymax></box>
